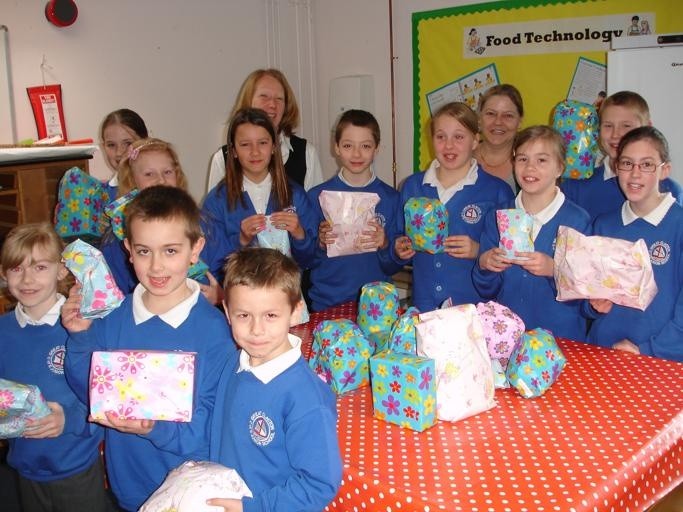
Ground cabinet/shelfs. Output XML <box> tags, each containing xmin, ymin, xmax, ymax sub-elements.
<box><xmin>0</xmin><ymin>154</ymin><xmax>94</xmax><ymax>312</ymax></box>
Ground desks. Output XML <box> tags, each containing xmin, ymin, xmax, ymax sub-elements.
<box><xmin>284</xmin><ymin>291</ymin><xmax>681</xmax><ymax>512</ymax></box>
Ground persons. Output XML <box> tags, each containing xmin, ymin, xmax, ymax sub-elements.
<box><xmin>197</xmin><ymin>108</ymin><xmax>319</xmax><ymax>287</ymax></box>
<box><xmin>555</xmin><ymin>91</ymin><xmax>681</xmax><ymax>220</ymax></box>
<box><xmin>467</xmin><ymin>84</ymin><xmax>522</xmax><ymax>199</ymax></box>
<box><xmin>207</xmin><ymin>69</ymin><xmax>323</xmax><ymax>197</ymax></box>
<box><xmin>59</xmin><ymin>185</ymin><xmax>238</xmax><ymax>511</ymax></box>
<box><xmin>305</xmin><ymin>109</ymin><xmax>401</xmax><ymax>314</ymax></box>
<box><xmin>100</xmin><ymin>110</ymin><xmax>149</xmax><ymax>194</ymax></box>
<box><xmin>579</xmin><ymin>126</ymin><xmax>682</xmax><ymax>364</ymax></box>
<box><xmin>207</xmin><ymin>247</ymin><xmax>343</xmax><ymax>512</ymax></box>
<box><xmin>100</xmin><ymin>139</ymin><xmax>225</xmax><ymax>306</ymax></box>
<box><xmin>0</xmin><ymin>220</ymin><xmax>100</xmax><ymax>510</ymax></box>
<box><xmin>472</xmin><ymin>127</ymin><xmax>589</xmax><ymax>345</ymax></box>
<box><xmin>379</xmin><ymin>103</ymin><xmax>515</xmax><ymax>318</ymax></box>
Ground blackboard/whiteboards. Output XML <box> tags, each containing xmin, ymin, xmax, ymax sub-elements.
<box><xmin>606</xmin><ymin>43</ymin><xmax>683</xmax><ymax>186</ymax></box>
